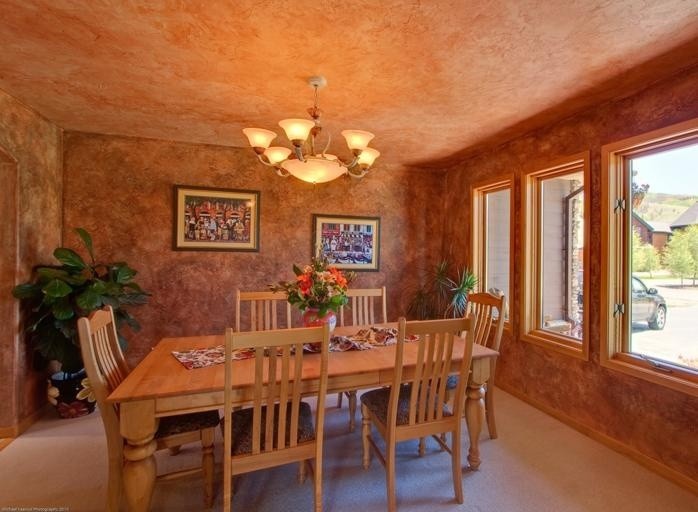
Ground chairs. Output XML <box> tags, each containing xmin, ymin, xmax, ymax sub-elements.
<box><xmin>77</xmin><ymin>285</ymin><xmax>508</xmax><ymax>512</ymax></box>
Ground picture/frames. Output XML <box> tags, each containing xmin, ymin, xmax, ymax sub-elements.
<box><xmin>172</xmin><ymin>183</ymin><xmax>381</xmax><ymax>273</ymax></box>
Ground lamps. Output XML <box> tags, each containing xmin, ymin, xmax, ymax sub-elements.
<box><xmin>240</xmin><ymin>76</ymin><xmax>380</xmax><ymax>190</ymax></box>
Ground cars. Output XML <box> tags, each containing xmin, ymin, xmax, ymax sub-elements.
<box><xmin>578</xmin><ymin>269</ymin><xmax>669</xmax><ymax>332</ymax></box>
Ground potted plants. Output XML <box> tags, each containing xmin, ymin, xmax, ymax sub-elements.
<box><xmin>12</xmin><ymin>226</ymin><xmax>153</xmax><ymax>421</ymax></box>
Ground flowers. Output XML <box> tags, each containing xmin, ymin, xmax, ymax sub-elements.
<box><xmin>267</xmin><ymin>244</ymin><xmax>359</xmax><ymax>319</ymax></box>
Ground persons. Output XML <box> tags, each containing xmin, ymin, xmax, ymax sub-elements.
<box><xmin>322</xmin><ymin>232</ymin><xmax>363</xmax><ymax>252</ymax></box>
<box><xmin>184</xmin><ymin>215</ymin><xmax>251</xmax><ymax>241</ymax></box>
